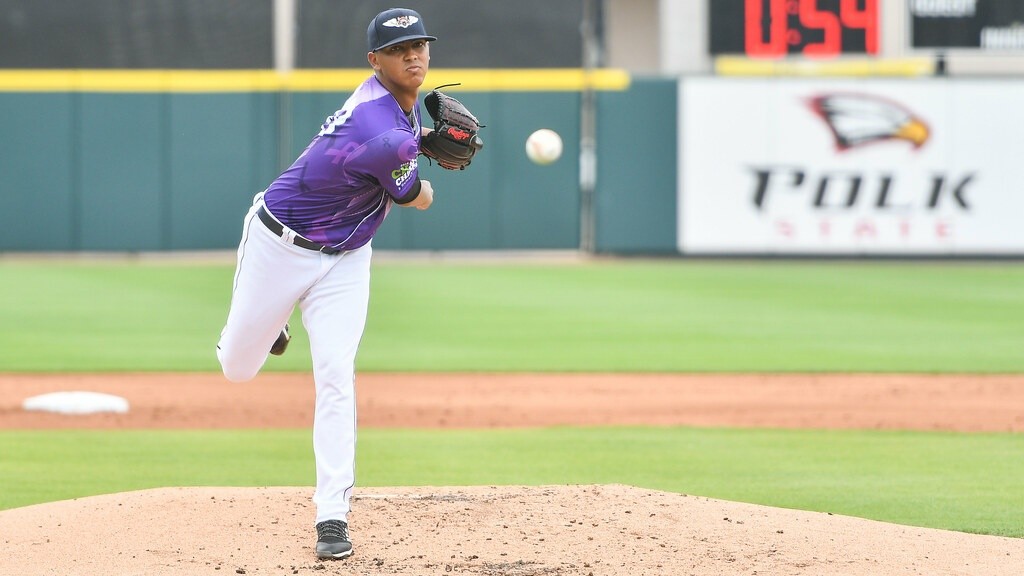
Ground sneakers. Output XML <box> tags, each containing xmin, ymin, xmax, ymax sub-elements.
<box><xmin>270</xmin><ymin>323</ymin><xmax>291</xmax><ymax>355</ymax></box>
<box><xmin>315</xmin><ymin>519</ymin><xmax>353</xmax><ymax>559</ymax></box>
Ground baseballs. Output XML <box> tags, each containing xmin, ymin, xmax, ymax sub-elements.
<box><xmin>527</xmin><ymin>129</ymin><xmax>564</xmax><ymax>166</ymax></box>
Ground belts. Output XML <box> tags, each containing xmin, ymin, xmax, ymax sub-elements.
<box><xmin>255</xmin><ymin>197</ymin><xmax>343</xmax><ymax>255</ymax></box>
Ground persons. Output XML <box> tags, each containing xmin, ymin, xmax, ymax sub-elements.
<box><xmin>214</xmin><ymin>6</ymin><xmax>485</xmax><ymax>559</ymax></box>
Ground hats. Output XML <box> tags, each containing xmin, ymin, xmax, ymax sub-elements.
<box><xmin>367</xmin><ymin>8</ymin><xmax>437</xmax><ymax>52</ymax></box>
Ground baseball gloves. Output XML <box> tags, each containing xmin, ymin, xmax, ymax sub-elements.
<box><xmin>422</xmin><ymin>92</ymin><xmax>485</xmax><ymax>169</ymax></box>
